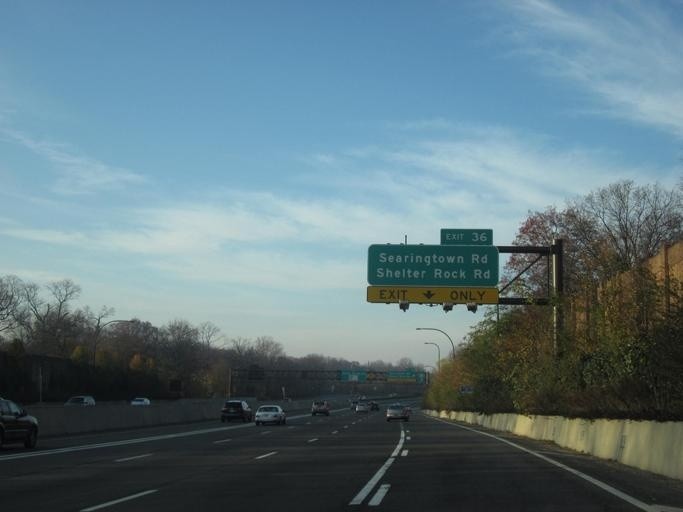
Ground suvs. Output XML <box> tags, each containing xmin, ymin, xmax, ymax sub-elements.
<box><xmin>221</xmin><ymin>399</ymin><xmax>252</xmax><ymax>423</ymax></box>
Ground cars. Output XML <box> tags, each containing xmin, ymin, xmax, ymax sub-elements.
<box><xmin>64</xmin><ymin>396</ymin><xmax>97</xmax><ymax>406</ymax></box>
<box><xmin>0</xmin><ymin>399</ymin><xmax>39</xmax><ymax>449</ymax></box>
<box><xmin>386</xmin><ymin>404</ymin><xmax>409</xmax><ymax>422</ymax></box>
<box><xmin>311</xmin><ymin>400</ymin><xmax>329</xmax><ymax>415</ymax></box>
<box><xmin>351</xmin><ymin>400</ymin><xmax>378</xmax><ymax>413</ymax></box>
<box><xmin>255</xmin><ymin>406</ymin><xmax>286</xmax><ymax>425</ymax></box>
<box><xmin>131</xmin><ymin>397</ymin><xmax>151</xmax><ymax>406</ymax></box>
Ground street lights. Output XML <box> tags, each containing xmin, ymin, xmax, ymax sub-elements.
<box><xmin>425</xmin><ymin>341</ymin><xmax>441</xmax><ymax>362</ymax></box>
<box><xmin>415</xmin><ymin>327</ymin><xmax>454</xmax><ymax>357</ymax></box>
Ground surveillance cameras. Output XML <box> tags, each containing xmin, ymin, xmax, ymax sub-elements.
<box><xmin>398</xmin><ymin>299</ymin><xmax>410</xmax><ymax>310</ymax></box>
<box><xmin>465</xmin><ymin>301</ymin><xmax>477</xmax><ymax>312</ymax></box>
<box><xmin>443</xmin><ymin>301</ymin><xmax>453</xmax><ymax>312</ymax></box>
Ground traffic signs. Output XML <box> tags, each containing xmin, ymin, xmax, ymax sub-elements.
<box><xmin>389</xmin><ymin>370</ymin><xmax>416</xmax><ymax>378</ymax></box>
<box><xmin>367</xmin><ymin>244</ymin><xmax>499</xmax><ymax>285</ymax></box>
<box><xmin>341</xmin><ymin>370</ymin><xmax>367</xmax><ymax>382</ymax></box>
